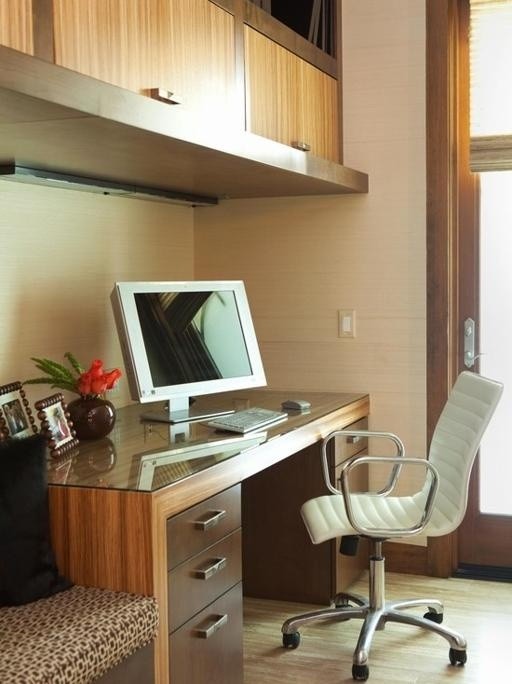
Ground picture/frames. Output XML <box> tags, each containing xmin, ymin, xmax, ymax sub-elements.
<box><xmin>0</xmin><ymin>380</ymin><xmax>37</xmax><ymax>440</ymax></box>
<box><xmin>34</xmin><ymin>393</ymin><xmax>80</xmax><ymax>457</ymax></box>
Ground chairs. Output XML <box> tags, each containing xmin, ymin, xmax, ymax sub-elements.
<box><xmin>282</xmin><ymin>371</ymin><xmax>505</xmax><ymax>682</ymax></box>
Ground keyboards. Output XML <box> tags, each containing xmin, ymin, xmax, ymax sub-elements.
<box><xmin>207</xmin><ymin>407</ymin><xmax>289</xmax><ymax>434</ymax></box>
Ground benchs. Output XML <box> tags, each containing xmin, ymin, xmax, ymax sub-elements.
<box><xmin>0</xmin><ymin>583</ymin><xmax>157</xmax><ymax>684</ymax></box>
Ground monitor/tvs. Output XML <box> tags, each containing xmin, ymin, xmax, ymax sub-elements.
<box><xmin>108</xmin><ymin>279</ymin><xmax>269</xmax><ymax>426</ymax></box>
<box><xmin>127</xmin><ymin>433</ymin><xmax>268</xmax><ymax>491</ymax></box>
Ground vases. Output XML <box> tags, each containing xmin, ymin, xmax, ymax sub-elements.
<box><xmin>68</xmin><ymin>400</ymin><xmax>115</xmax><ymax>437</ymax></box>
<box><xmin>72</xmin><ymin>437</ymin><xmax>116</xmax><ymax>476</ymax></box>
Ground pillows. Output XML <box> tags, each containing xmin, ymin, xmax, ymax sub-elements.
<box><xmin>1</xmin><ymin>434</ymin><xmax>75</xmax><ymax>609</ymax></box>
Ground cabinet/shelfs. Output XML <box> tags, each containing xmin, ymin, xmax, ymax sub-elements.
<box><xmin>43</xmin><ymin>389</ymin><xmax>371</xmax><ymax>684</ymax></box>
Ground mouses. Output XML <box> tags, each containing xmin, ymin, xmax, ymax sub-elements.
<box><xmin>283</xmin><ymin>399</ymin><xmax>312</xmax><ymax>411</ymax></box>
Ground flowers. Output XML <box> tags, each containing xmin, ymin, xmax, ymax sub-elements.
<box><xmin>23</xmin><ymin>352</ymin><xmax>122</xmax><ymax>399</ymax></box>
